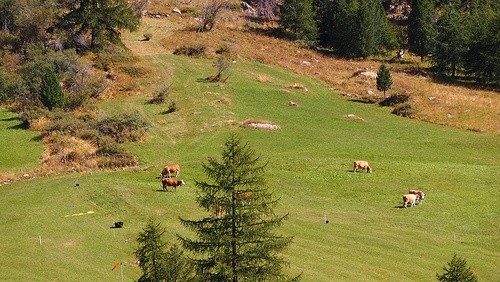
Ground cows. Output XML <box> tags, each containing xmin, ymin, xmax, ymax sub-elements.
<box><xmin>403</xmin><ymin>194</ymin><xmax>420</xmax><ymax>208</ymax></box>
<box><xmin>162</xmin><ymin>177</ymin><xmax>185</xmax><ymax>192</ymax></box>
<box><xmin>409</xmin><ymin>190</ymin><xmax>425</xmax><ymax>205</ymax></box>
<box><xmin>354</xmin><ymin>161</ymin><xmax>372</xmax><ymax>173</ymax></box>
<box><xmin>160</xmin><ymin>163</ymin><xmax>180</xmax><ymax>178</ymax></box>
